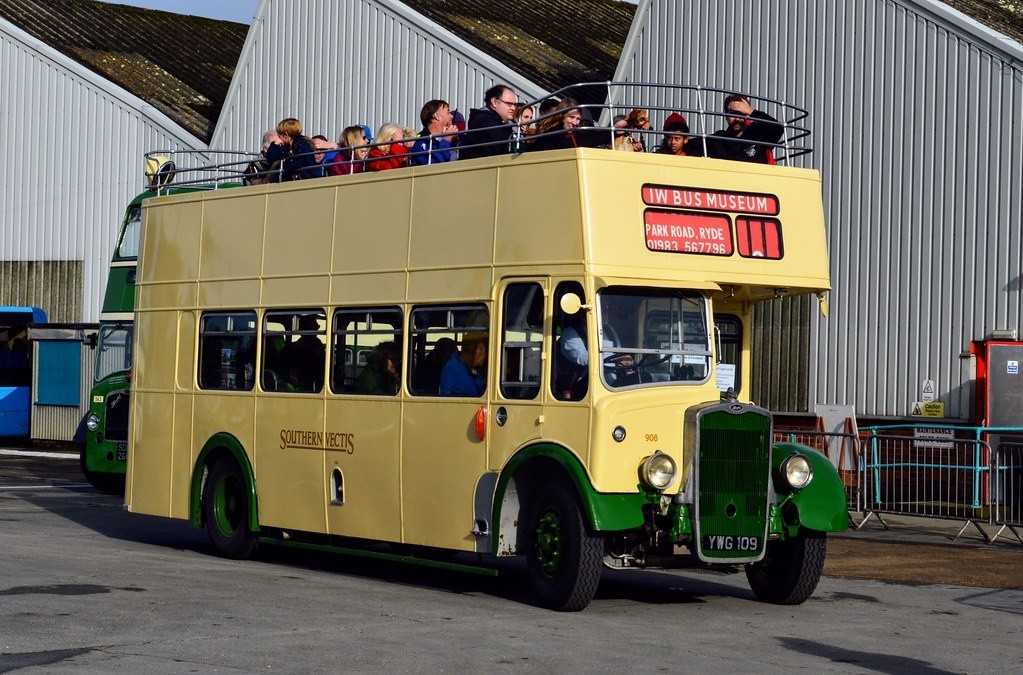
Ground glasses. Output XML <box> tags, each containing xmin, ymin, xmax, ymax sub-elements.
<box><xmin>496</xmin><ymin>97</ymin><xmax>517</xmax><ymax>109</ymax></box>
<box><xmin>601</xmin><ymin>306</ymin><xmax>610</xmax><ymax>313</ymax></box>
<box><xmin>384</xmin><ymin>357</ymin><xmax>402</xmax><ymax>364</ymax></box>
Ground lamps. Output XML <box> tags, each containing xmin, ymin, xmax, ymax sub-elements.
<box><xmin>960</xmin><ymin>351</ymin><xmax>971</xmax><ymax>359</ymax></box>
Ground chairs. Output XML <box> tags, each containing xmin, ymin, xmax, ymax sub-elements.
<box><xmin>248</xmin><ymin>329</ymin><xmax>301</xmax><ymax>392</ymax></box>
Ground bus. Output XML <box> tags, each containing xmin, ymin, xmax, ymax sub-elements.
<box><xmin>84</xmin><ymin>79</ymin><xmax>848</xmax><ymax>612</ymax></box>
<box><xmin>0</xmin><ymin>306</ymin><xmax>50</xmax><ymax>440</ymax></box>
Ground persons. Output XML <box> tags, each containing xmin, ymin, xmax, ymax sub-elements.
<box><xmin>239</xmin><ymin>84</ymin><xmax>784</xmax><ymax>164</ymax></box>
<box><xmin>204</xmin><ymin>295</ymin><xmax>636</xmax><ymax>403</ymax></box>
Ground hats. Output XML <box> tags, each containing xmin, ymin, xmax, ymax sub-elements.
<box><xmin>664</xmin><ymin>113</ymin><xmax>684</xmax><ymax>129</ymax></box>
<box><xmin>461</xmin><ymin>332</ymin><xmax>489</xmax><ymax>350</ymax></box>
<box><xmin>357</xmin><ymin>125</ymin><xmax>373</xmax><ymax>140</ymax></box>
<box><xmin>451</xmin><ymin>110</ymin><xmax>464</xmax><ymax>131</ymax></box>
